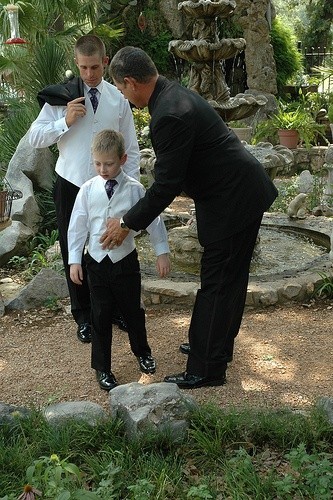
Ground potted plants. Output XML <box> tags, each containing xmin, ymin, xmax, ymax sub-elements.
<box><xmin>228</xmin><ymin>120</ymin><xmax>252</xmax><ymax>142</ymax></box>
<box><xmin>253</xmin><ymin>74</ymin><xmax>330</xmax><ymax>154</ymax></box>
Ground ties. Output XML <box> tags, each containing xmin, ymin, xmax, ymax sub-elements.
<box><xmin>89</xmin><ymin>87</ymin><xmax>99</xmax><ymax>115</ymax></box>
<box><xmin>105</xmin><ymin>180</ymin><xmax>117</xmax><ymax>200</ymax></box>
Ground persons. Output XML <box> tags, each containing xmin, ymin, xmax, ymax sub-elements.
<box><xmin>29</xmin><ymin>35</ymin><xmax>141</xmax><ymax>343</ymax></box>
<box><xmin>98</xmin><ymin>47</ymin><xmax>279</xmax><ymax>390</ymax></box>
<box><xmin>68</xmin><ymin>129</ymin><xmax>173</xmax><ymax>389</ymax></box>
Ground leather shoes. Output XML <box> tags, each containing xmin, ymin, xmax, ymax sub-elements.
<box><xmin>95</xmin><ymin>369</ymin><xmax>117</xmax><ymax>390</ymax></box>
<box><xmin>164</xmin><ymin>368</ymin><xmax>228</xmax><ymax>390</ymax></box>
<box><xmin>134</xmin><ymin>350</ymin><xmax>155</xmax><ymax>373</ymax></box>
<box><xmin>112</xmin><ymin>312</ymin><xmax>128</xmax><ymax>331</ymax></box>
<box><xmin>179</xmin><ymin>343</ymin><xmax>190</xmax><ymax>355</ymax></box>
<box><xmin>77</xmin><ymin>319</ymin><xmax>92</xmax><ymax>343</ymax></box>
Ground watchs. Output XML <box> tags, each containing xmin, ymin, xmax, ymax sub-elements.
<box><xmin>120</xmin><ymin>218</ymin><xmax>130</xmax><ymax>232</ymax></box>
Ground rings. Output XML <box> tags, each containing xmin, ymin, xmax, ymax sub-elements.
<box><xmin>111</xmin><ymin>241</ymin><xmax>116</xmax><ymax>246</ymax></box>
